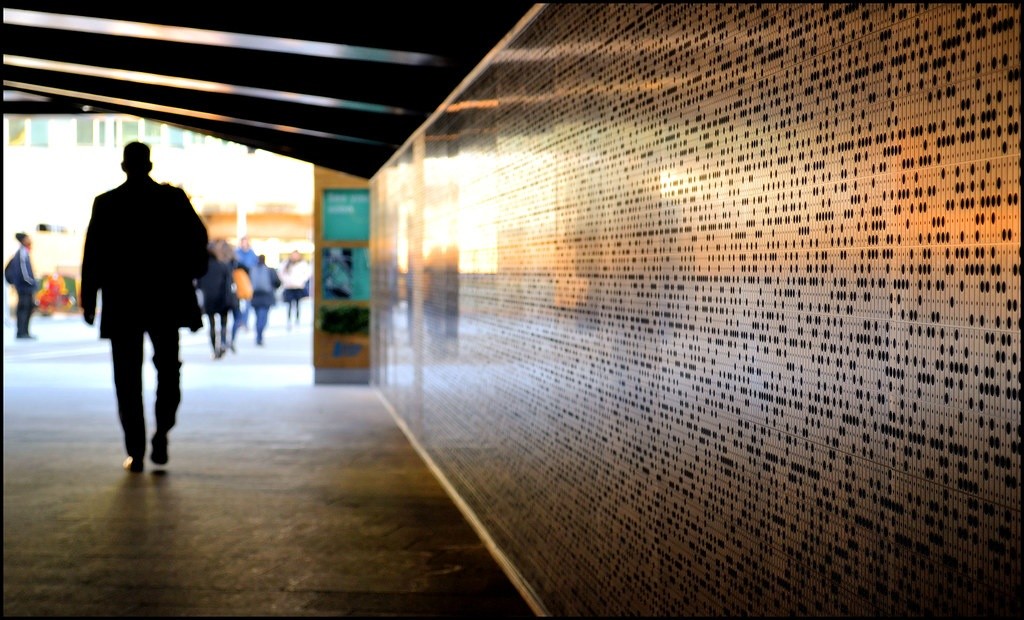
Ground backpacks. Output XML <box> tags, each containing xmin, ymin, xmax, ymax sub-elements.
<box><xmin>5</xmin><ymin>257</ymin><xmax>16</xmax><ymax>284</ymax></box>
<box><xmin>250</xmin><ymin>266</ymin><xmax>272</xmax><ymax>293</ymax></box>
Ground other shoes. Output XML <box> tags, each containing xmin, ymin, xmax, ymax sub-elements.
<box><xmin>124</xmin><ymin>456</ymin><xmax>143</xmax><ymax>472</ymax></box>
<box><xmin>150</xmin><ymin>433</ymin><xmax>168</xmax><ymax>465</ymax></box>
<box><xmin>16</xmin><ymin>334</ymin><xmax>35</xmax><ymax>339</ymax></box>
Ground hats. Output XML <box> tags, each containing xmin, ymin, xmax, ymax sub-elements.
<box><xmin>16</xmin><ymin>233</ymin><xmax>26</xmax><ymax>242</ymax></box>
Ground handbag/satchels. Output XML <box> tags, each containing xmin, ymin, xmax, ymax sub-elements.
<box><xmin>232</xmin><ymin>268</ymin><xmax>253</xmax><ymax>301</ymax></box>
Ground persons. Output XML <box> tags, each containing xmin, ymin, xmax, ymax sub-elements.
<box><xmin>202</xmin><ymin>236</ymin><xmax>314</xmax><ymax>358</ymax></box>
<box><xmin>80</xmin><ymin>142</ymin><xmax>211</xmax><ymax>472</ymax></box>
<box><xmin>6</xmin><ymin>232</ymin><xmax>41</xmax><ymax>337</ymax></box>
